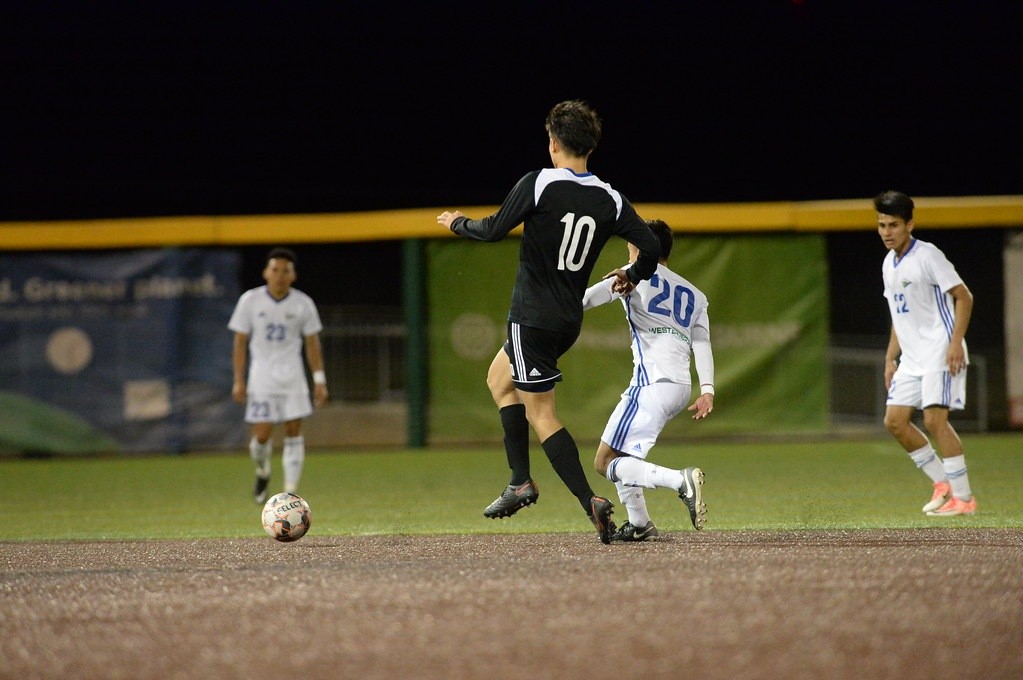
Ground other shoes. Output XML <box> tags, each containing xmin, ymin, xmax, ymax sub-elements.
<box><xmin>254</xmin><ymin>475</ymin><xmax>269</xmax><ymax>503</ymax></box>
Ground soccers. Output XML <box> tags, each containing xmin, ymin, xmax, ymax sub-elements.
<box><xmin>261</xmin><ymin>493</ymin><xmax>312</xmax><ymax>542</ymax></box>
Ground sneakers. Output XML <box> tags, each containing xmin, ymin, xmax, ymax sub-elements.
<box><xmin>589</xmin><ymin>495</ymin><xmax>615</xmax><ymax>545</ymax></box>
<box><xmin>612</xmin><ymin>520</ymin><xmax>659</xmax><ymax>544</ymax></box>
<box><xmin>922</xmin><ymin>480</ymin><xmax>951</xmax><ymax>512</ymax></box>
<box><xmin>678</xmin><ymin>467</ymin><xmax>708</xmax><ymax>530</ymax></box>
<box><xmin>927</xmin><ymin>496</ymin><xmax>975</xmax><ymax>516</ymax></box>
<box><xmin>484</xmin><ymin>477</ymin><xmax>539</xmax><ymax>520</ymax></box>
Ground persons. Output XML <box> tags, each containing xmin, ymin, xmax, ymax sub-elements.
<box><xmin>582</xmin><ymin>218</ymin><xmax>717</xmax><ymax>540</ymax></box>
<box><xmin>226</xmin><ymin>253</ymin><xmax>328</xmax><ymax>503</ymax></box>
<box><xmin>436</xmin><ymin>98</ymin><xmax>660</xmax><ymax>545</ymax></box>
<box><xmin>872</xmin><ymin>191</ymin><xmax>977</xmax><ymax>516</ymax></box>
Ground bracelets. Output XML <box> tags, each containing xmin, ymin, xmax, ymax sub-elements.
<box><xmin>313</xmin><ymin>369</ymin><xmax>326</xmax><ymax>385</ymax></box>
<box><xmin>701</xmin><ymin>385</ymin><xmax>715</xmax><ymax>396</ymax></box>
